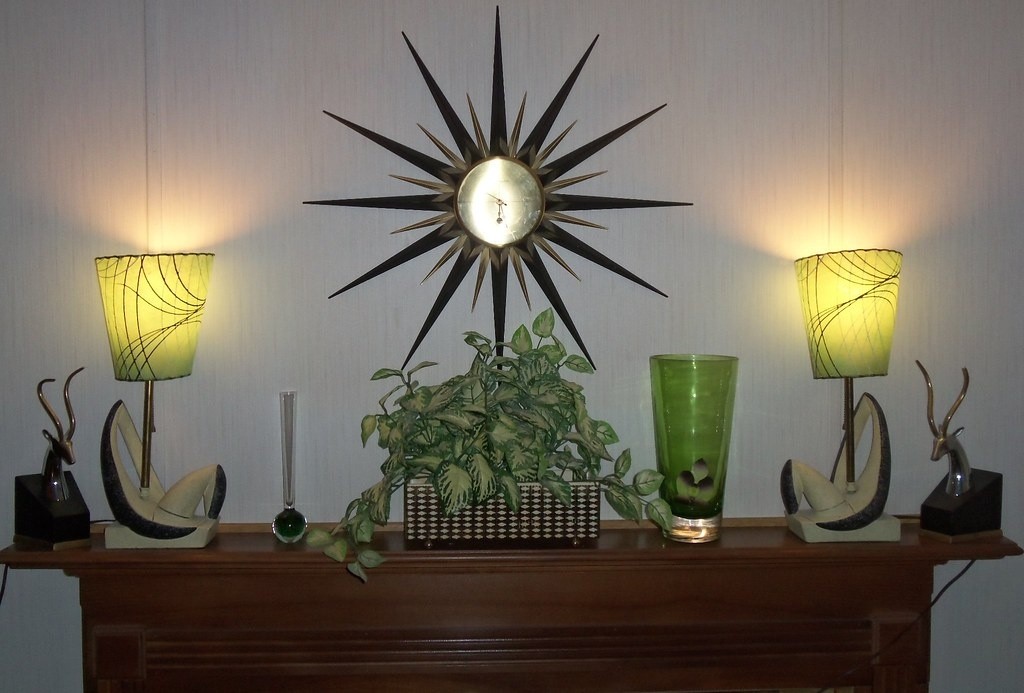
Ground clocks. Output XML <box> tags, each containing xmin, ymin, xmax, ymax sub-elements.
<box><xmin>302</xmin><ymin>5</ymin><xmax>695</xmax><ymax>391</ymax></box>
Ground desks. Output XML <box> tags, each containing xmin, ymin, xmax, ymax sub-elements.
<box><xmin>0</xmin><ymin>514</ymin><xmax>1024</xmax><ymax>693</ymax></box>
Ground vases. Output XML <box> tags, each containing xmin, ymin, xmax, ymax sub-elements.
<box><xmin>272</xmin><ymin>386</ymin><xmax>308</xmax><ymax>550</ymax></box>
<box><xmin>650</xmin><ymin>352</ymin><xmax>739</xmax><ymax>546</ymax></box>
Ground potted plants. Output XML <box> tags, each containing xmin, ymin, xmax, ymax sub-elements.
<box><xmin>303</xmin><ymin>306</ymin><xmax>692</xmax><ymax>583</ymax></box>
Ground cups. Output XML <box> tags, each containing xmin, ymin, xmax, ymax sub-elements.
<box><xmin>649</xmin><ymin>354</ymin><xmax>739</xmax><ymax>544</ymax></box>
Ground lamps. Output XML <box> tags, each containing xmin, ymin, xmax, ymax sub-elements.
<box><xmin>776</xmin><ymin>248</ymin><xmax>905</xmax><ymax>546</ymax></box>
<box><xmin>97</xmin><ymin>251</ymin><xmax>227</xmax><ymax>553</ymax></box>
